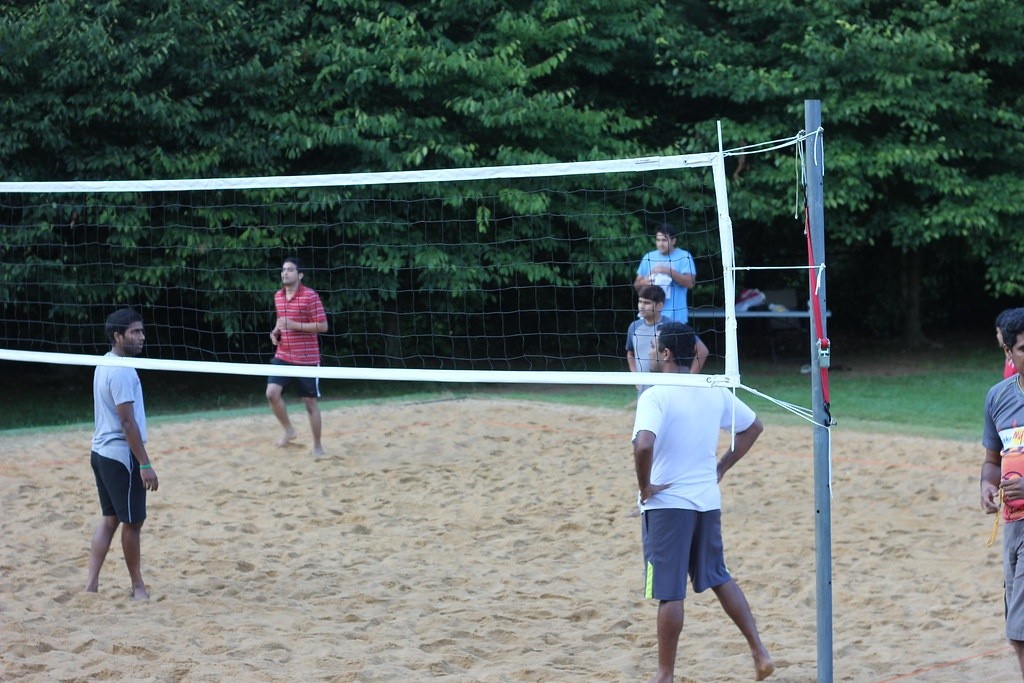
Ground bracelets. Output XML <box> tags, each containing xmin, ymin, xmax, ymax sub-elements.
<box><xmin>140</xmin><ymin>463</ymin><xmax>151</xmax><ymax>469</ymax></box>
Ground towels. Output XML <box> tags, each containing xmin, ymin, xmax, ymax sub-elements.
<box><xmin>650</xmin><ymin>273</ymin><xmax>672</xmax><ymax>300</ymax></box>
<box><xmin>735</xmin><ymin>287</ymin><xmax>767</xmax><ymax>314</ymax></box>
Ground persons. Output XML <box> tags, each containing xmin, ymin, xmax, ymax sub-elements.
<box><xmin>82</xmin><ymin>309</ymin><xmax>158</xmax><ymax>600</ymax></box>
<box><xmin>264</xmin><ymin>260</ymin><xmax>329</xmax><ymax>455</ymax></box>
<box><xmin>979</xmin><ymin>308</ymin><xmax>1024</xmax><ymax>678</ymax></box>
<box><xmin>626</xmin><ymin>286</ymin><xmax>709</xmax><ymax>517</ymax></box>
<box><xmin>632</xmin><ymin>322</ymin><xmax>775</xmax><ymax>683</ymax></box>
<box><xmin>634</xmin><ymin>226</ymin><xmax>695</xmax><ymax>330</ymax></box>
